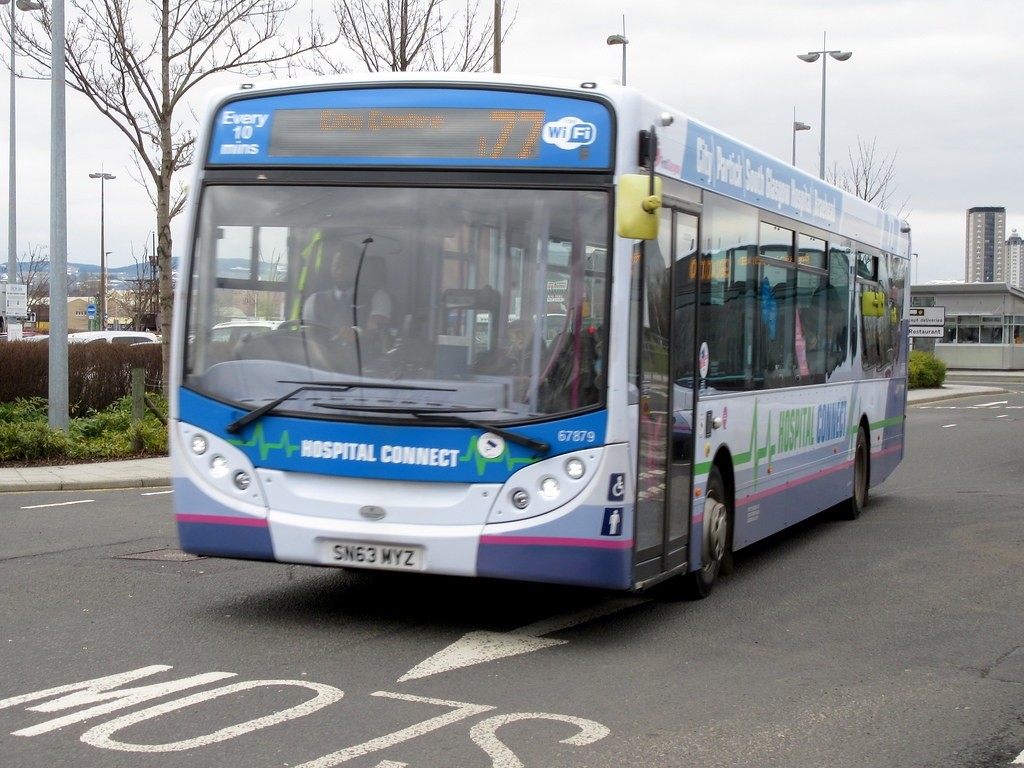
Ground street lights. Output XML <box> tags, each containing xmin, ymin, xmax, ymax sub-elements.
<box><xmin>0</xmin><ymin>0</ymin><xmax>17</xmax><ymax>324</ymax></box>
<box><xmin>89</xmin><ymin>162</ymin><xmax>117</xmax><ymax>331</ymax></box>
<box><xmin>792</xmin><ymin>106</ymin><xmax>810</xmax><ymax>167</ymax></box>
<box><xmin>912</xmin><ymin>252</ymin><xmax>918</xmax><ymax>285</ymax></box>
<box><xmin>105</xmin><ymin>251</ymin><xmax>112</xmax><ymax>331</ymax></box>
<box><xmin>607</xmin><ymin>34</ymin><xmax>629</xmax><ymax>87</ymax></box>
<box><xmin>16</xmin><ymin>0</ymin><xmax>70</xmax><ymax>434</ymax></box>
<box><xmin>797</xmin><ymin>51</ymin><xmax>853</xmax><ymax>181</ymax></box>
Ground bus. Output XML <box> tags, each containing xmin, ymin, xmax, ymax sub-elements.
<box><xmin>169</xmin><ymin>73</ymin><xmax>912</xmax><ymax>604</ymax></box>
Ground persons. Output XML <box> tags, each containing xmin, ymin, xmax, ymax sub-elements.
<box><xmin>302</xmin><ymin>241</ymin><xmax>392</xmax><ymax>357</ymax></box>
<box><xmin>503</xmin><ymin>320</ymin><xmax>546</xmax><ymax>378</ymax></box>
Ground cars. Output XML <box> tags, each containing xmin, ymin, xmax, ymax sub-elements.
<box><xmin>212</xmin><ymin>320</ymin><xmax>282</xmax><ymax>341</ymax></box>
<box><xmin>69</xmin><ymin>331</ymin><xmax>157</xmax><ymax>345</ymax></box>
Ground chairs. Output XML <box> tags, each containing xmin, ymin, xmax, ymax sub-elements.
<box><xmin>302</xmin><ymin>225</ymin><xmax>892</xmax><ymax>401</ymax></box>
